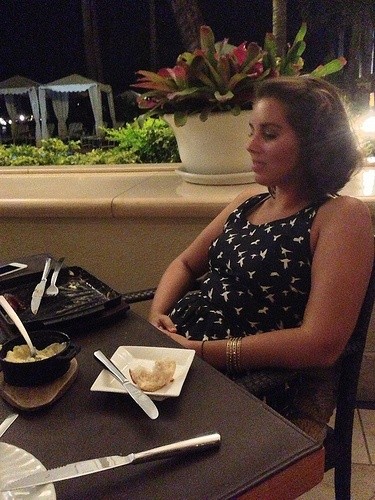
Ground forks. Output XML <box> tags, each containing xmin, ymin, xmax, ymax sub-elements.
<box><xmin>45</xmin><ymin>258</ymin><xmax>64</xmax><ymax>297</ymax></box>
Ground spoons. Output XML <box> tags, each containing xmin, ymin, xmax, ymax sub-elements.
<box><xmin>0</xmin><ymin>295</ymin><xmax>38</xmax><ymax>358</ymax></box>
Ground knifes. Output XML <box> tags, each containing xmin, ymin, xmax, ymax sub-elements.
<box><xmin>31</xmin><ymin>257</ymin><xmax>52</xmax><ymax>316</ymax></box>
<box><xmin>0</xmin><ymin>433</ymin><xmax>221</xmax><ymax>491</ymax></box>
<box><xmin>94</xmin><ymin>349</ymin><xmax>159</xmax><ymax>420</ymax></box>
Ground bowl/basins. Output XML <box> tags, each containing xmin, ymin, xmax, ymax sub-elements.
<box><xmin>0</xmin><ymin>330</ymin><xmax>81</xmax><ymax>387</ymax></box>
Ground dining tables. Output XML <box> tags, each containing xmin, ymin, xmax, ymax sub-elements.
<box><xmin>0</xmin><ymin>251</ymin><xmax>325</xmax><ymax>500</ymax></box>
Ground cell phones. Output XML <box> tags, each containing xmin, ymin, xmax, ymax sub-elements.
<box><xmin>0</xmin><ymin>263</ymin><xmax>28</xmax><ymax>277</ymax></box>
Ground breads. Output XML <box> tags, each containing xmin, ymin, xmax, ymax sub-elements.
<box><xmin>129</xmin><ymin>359</ymin><xmax>176</xmax><ymax>392</ymax></box>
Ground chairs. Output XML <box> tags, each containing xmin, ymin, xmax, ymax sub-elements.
<box><xmin>121</xmin><ymin>261</ymin><xmax>375</xmax><ymax>500</ymax></box>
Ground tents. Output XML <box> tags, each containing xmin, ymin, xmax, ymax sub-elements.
<box><xmin>39</xmin><ymin>74</ymin><xmax>116</xmax><ymax>148</ymax></box>
<box><xmin>0</xmin><ymin>75</ymin><xmax>41</xmax><ymax>148</ymax></box>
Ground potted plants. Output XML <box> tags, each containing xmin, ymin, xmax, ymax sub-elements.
<box><xmin>131</xmin><ymin>24</ymin><xmax>347</xmax><ymax>186</ymax></box>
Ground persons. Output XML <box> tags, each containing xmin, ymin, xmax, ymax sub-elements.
<box><xmin>143</xmin><ymin>76</ymin><xmax>374</xmax><ymax>443</ymax></box>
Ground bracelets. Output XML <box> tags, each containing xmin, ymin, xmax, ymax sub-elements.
<box><xmin>225</xmin><ymin>336</ymin><xmax>243</xmax><ymax>373</ymax></box>
<box><xmin>200</xmin><ymin>338</ymin><xmax>208</xmax><ymax>359</ymax></box>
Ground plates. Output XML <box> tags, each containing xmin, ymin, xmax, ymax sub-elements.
<box><xmin>0</xmin><ymin>442</ymin><xmax>56</xmax><ymax>499</ymax></box>
<box><xmin>90</xmin><ymin>346</ymin><xmax>196</xmax><ymax>402</ymax></box>
